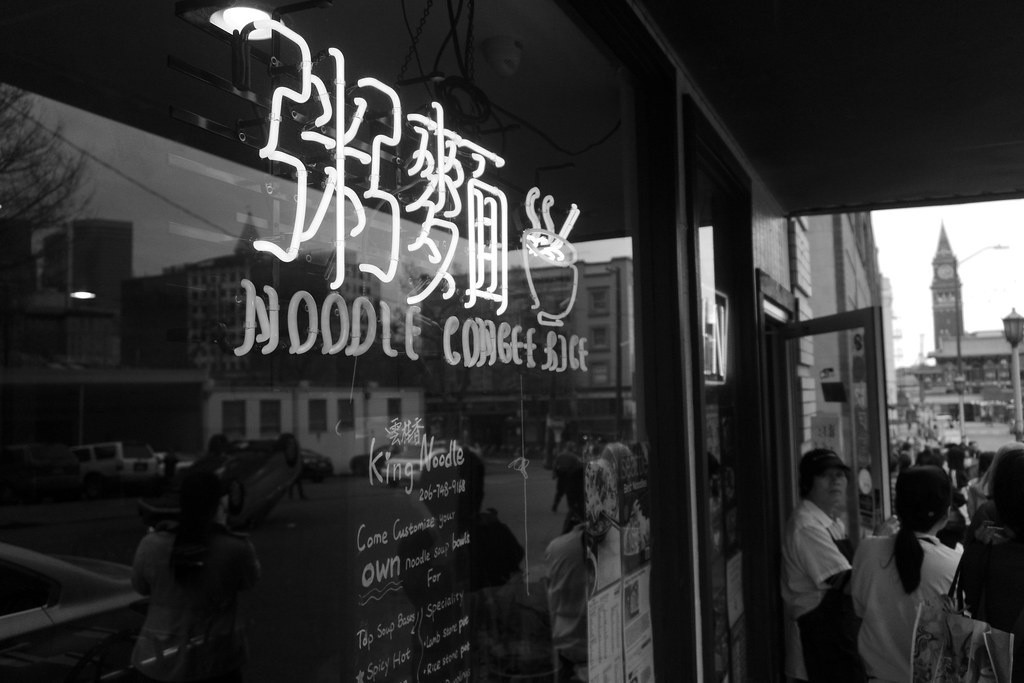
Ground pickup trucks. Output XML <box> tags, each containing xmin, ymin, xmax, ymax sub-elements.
<box><xmin>68</xmin><ymin>440</ymin><xmax>166</xmax><ymax>497</ymax></box>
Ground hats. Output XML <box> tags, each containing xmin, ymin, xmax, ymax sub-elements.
<box><xmin>800</xmin><ymin>448</ymin><xmax>850</xmax><ymax>476</ymax></box>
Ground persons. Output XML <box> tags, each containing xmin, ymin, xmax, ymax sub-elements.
<box><xmin>128</xmin><ymin>430</ymin><xmax>730</xmax><ymax>683</ymax></box>
<box><xmin>780</xmin><ymin>423</ymin><xmax>1024</xmax><ymax>683</ymax></box>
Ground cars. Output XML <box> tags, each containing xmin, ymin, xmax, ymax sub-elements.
<box><xmin>380</xmin><ymin>440</ymin><xmax>485</xmax><ymax>488</ymax></box>
<box><xmin>227</xmin><ymin>438</ymin><xmax>332</xmax><ymax>484</ymax></box>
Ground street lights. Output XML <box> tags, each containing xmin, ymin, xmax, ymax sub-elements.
<box><xmin>953</xmin><ymin>245</ymin><xmax>1010</xmax><ymax>445</ymax></box>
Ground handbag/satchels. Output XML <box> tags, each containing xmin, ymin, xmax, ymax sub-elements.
<box><xmin>909</xmin><ymin>537</ymin><xmax>1015</xmax><ymax>683</ymax></box>
